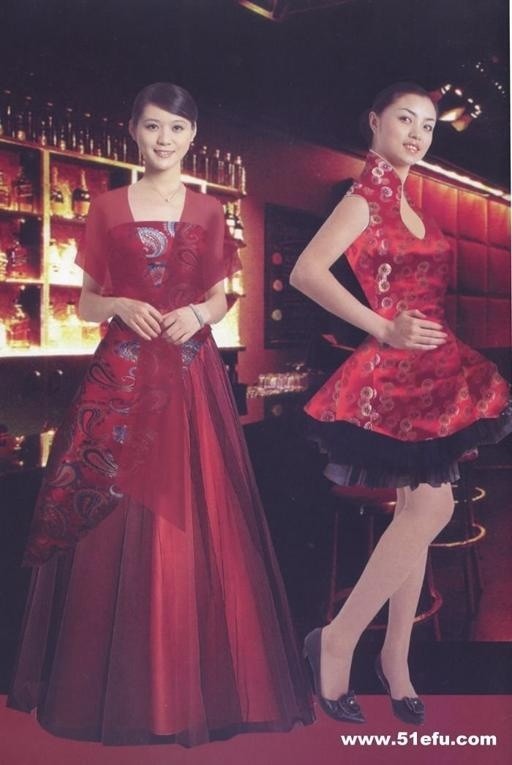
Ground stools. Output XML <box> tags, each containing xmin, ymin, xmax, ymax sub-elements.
<box><xmin>325</xmin><ymin>444</ymin><xmax>486</xmax><ymax>642</ymax></box>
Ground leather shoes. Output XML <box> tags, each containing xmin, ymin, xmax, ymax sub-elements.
<box><xmin>373</xmin><ymin>649</ymin><xmax>426</xmax><ymax>725</ymax></box>
<box><xmin>301</xmin><ymin>627</ymin><xmax>365</xmax><ymax>723</ymax></box>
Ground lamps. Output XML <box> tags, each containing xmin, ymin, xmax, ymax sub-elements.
<box><xmin>437</xmin><ymin>83</ymin><xmax>483</xmax><ymax>133</ymax></box>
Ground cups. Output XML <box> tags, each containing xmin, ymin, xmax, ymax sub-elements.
<box><xmin>39</xmin><ymin>432</ymin><xmax>56</xmax><ymax>467</ymax></box>
<box><xmin>247</xmin><ymin>370</ymin><xmax>308</xmax><ymax>401</ymax></box>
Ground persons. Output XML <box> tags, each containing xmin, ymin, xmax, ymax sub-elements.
<box><xmin>6</xmin><ymin>81</ymin><xmax>316</xmax><ymax>747</ymax></box>
<box><xmin>288</xmin><ymin>78</ymin><xmax>511</xmax><ymax>727</ymax></box>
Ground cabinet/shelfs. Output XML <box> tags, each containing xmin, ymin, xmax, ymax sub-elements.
<box><xmin>0</xmin><ymin>140</ymin><xmax>247</xmax><ymax>357</ymax></box>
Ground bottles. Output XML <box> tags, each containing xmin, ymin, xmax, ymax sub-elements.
<box><xmin>6</xmin><ymin>234</ymin><xmax>28</xmax><ymax>279</ymax></box>
<box><xmin>49</xmin><ymin>300</ymin><xmax>82</xmax><ymax>345</ymax></box>
<box><xmin>0</xmin><ymin>166</ymin><xmax>36</xmax><ymax>210</ymax></box>
<box><xmin>6</xmin><ymin>299</ymin><xmax>32</xmax><ymax>349</ymax></box>
<box><xmin>51</xmin><ymin>167</ymin><xmax>64</xmax><ymax>217</ymax></box>
<box><xmin>50</xmin><ymin>239</ymin><xmax>77</xmax><ymax>280</ymax></box>
<box><xmin>225</xmin><ymin>202</ymin><xmax>244</xmax><ymax>242</ymax></box>
<box><xmin>0</xmin><ymin>87</ymin><xmax>246</xmax><ymax>192</ymax></box>
<box><xmin>226</xmin><ymin>266</ymin><xmax>247</xmax><ymax>296</ymax></box>
<box><xmin>71</xmin><ymin>167</ymin><xmax>92</xmax><ymax>221</ymax></box>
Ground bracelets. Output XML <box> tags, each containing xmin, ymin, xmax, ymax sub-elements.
<box><xmin>188</xmin><ymin>303</ymin><xmax>207</xmax><ymax>326</ymax></box>
